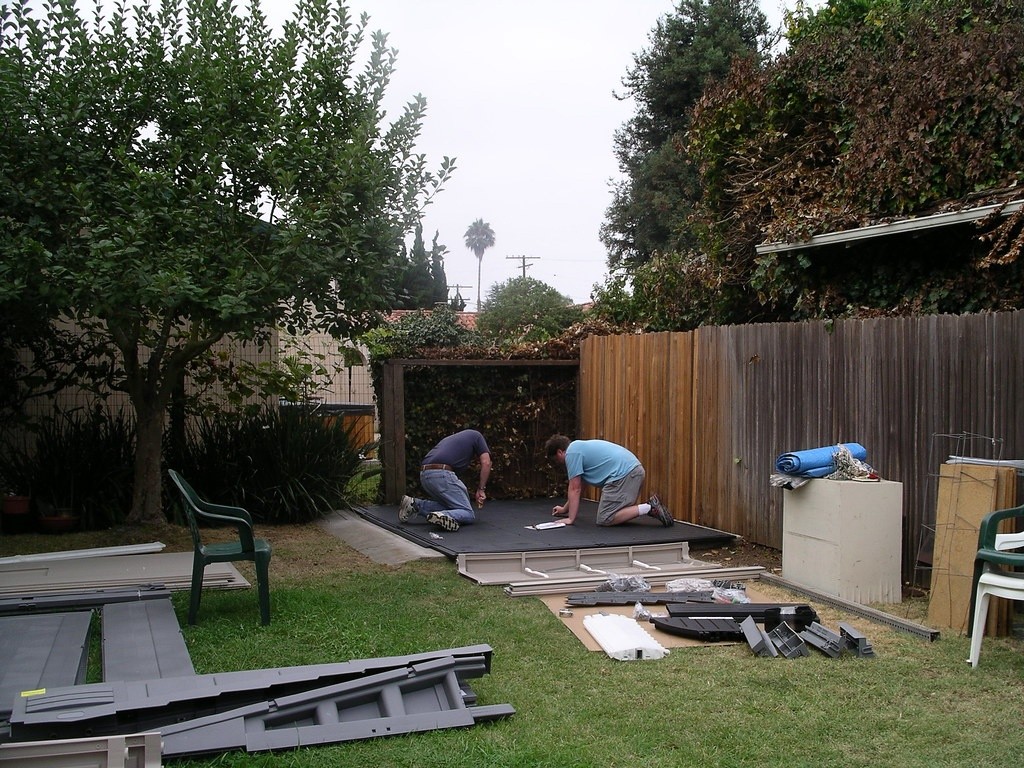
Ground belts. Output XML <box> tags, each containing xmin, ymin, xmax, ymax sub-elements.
<box><xmin>420</xmin><ymin>464</ymin><xmax>452</xmax><ymax>471</ymax></box>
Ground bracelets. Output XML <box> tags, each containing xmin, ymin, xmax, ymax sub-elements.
<box><xmin>478</xmin><ymin>486</ymin><xmax>486</xmax><ymax>490</ymax></box>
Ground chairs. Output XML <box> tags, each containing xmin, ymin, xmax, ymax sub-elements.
<box><xmin>167</xmin><ymin>468</ymin><xmax>273</xmax><ymax>627</ymax></box>
<box><xmin>971</xmin><ymin>504</ymin><xmax>1024</xmax><ymax>671</ymax></box>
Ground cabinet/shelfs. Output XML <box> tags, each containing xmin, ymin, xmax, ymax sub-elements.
<box><xmin>781</xmin><ymin>478</ymin><xmax>905</xmax><ymax>607</ymax></box>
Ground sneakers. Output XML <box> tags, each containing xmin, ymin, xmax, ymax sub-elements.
<box><xmin>426</xmin><ymin>511</ymin><xmax>459</xmax><ymax>532</ymax></box>
<box><xmin>399</xmin><ymin>495</ymin><xmax>420</xmax><ymax>523</ymax></box>
<box><xmin>644</xmin><ymin>494</ymin><xmax>673</xmax><ymax>526</ymax></box>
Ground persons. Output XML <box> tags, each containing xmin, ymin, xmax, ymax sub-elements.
<box><xmin>398</xmin><ymin>430</ymin><xmax>493</xmax><ymax>532</ymax></box>
<box><xmin>545</xmin><ymin>432</ymin><xmax>674</xmax><ymax>527</ymax></box>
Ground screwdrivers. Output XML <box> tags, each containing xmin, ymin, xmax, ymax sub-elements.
<box><xmin>530</xmin><ymin>521</ymin><xmax>542</xmax><ymax>534</ymax></box>
<box><xmin>477</xmin><ymin>497</ymin><xmax>482</xmax><ymax>520</ymax></box>
